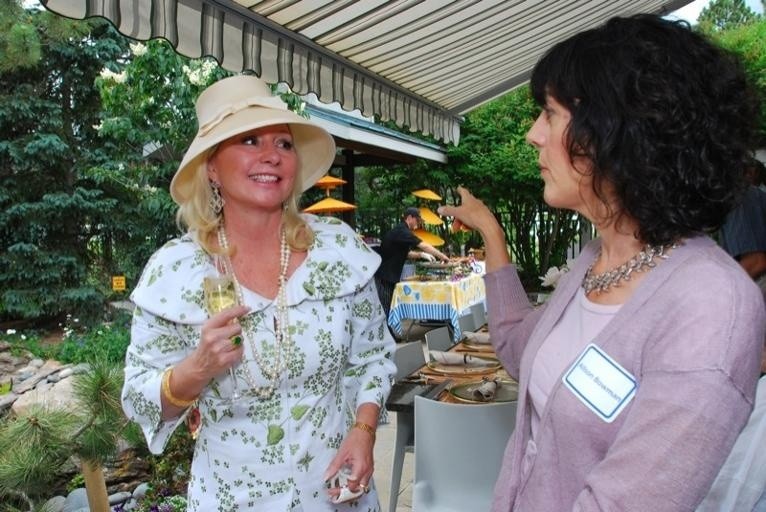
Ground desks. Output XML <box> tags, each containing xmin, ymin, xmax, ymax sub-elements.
<box><xmin>387</xmin><ymin>261</ymin><xmax>486</xmax><ymax>344</ymax></box>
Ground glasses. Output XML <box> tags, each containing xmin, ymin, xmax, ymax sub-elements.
<box><xmin>331</xmin><ymin>469</ymin><xmax>364</xmax><ymax>505</ymax></box>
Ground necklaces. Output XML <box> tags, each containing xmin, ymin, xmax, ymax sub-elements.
<box><xmin>216</xmin><ymin>221</ymin><xmax>288</xmax><ymax>399</ymax></box>
<box><xmin>582</xmin><ymin>241</ymin><xmax>686</xmax><ymax>295</ymax></box>
<box><xmin>219</xmin><ymin>225</ymin><xmax>294</xmax><ymax>376</ymax></box>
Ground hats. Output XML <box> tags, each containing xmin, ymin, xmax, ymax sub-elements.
<box><xmin>169</xmin><ymin>75</ymin><xmax>336</xmax><ymax>206</ymax></box>
<box><xmin>406</xmin><ymin>207</ymin><xmax>422</xmax><ymax>220</ymax></box>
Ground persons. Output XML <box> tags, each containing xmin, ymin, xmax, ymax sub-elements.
<box><xmin>118</xmin><ymin>72</ymin><xmax>400</xmax><ymax>512</ymax></box>
<box><xmin>373</xmin><ymin>207</ymin><xmax>449</xmax><ymax>343</ymax></box>
<box><xmin>713</xmin><ymin>159</ymin><xmax>766</xmax><ymax>298</ymax></box>
<box><xmin>435</xmin><ymin>11</ymin><xmax>766</xmax><ymax>512</ymax></box>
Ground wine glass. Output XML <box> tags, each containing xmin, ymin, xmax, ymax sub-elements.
<box><xmin>203</xmin><ymin>274</ymin><xmax>258</xmax><ymax>406</ymax></box>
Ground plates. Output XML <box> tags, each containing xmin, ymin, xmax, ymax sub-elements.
<box><xmin>448</xmin><ymin>379</ymin><xmax>520</xmax><ymax>405</ymax></box>
<box><xmin>427</xmin><ymin>354</ymin><xmax>502</xmax><ymax>377</ymax></box>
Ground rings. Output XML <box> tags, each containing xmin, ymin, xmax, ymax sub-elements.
<box><xmin>359</xmin><ymin>483</ymin><xmax>370</xmax><ymax>493</ymax></box>
<box><xmin>230</xmin><ymin>335</ymin><xmax>241</xmax><ymax>346</ymax></box>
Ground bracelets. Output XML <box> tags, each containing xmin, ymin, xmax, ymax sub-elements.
<box><xmin>351</xmin><ymin>422</ymin><xmax>375</xmax><ymax>438</ymax></box>
<box><xmin>161</xmin><ymin>370</ymin><xmax>199</xmax><ymax>409</ymax></box>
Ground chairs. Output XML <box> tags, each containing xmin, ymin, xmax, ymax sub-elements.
<box><xmin>388</xmin><ymin>303</ymin><xmax>517</xmax><ymax>512</ymax></box>
